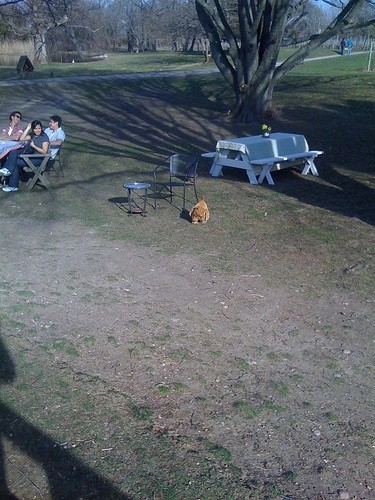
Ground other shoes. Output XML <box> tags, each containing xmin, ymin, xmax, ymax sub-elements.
<box><xmin>0</xmin><ymin>168</ymin><xmax>12</xmax><ymax>177</ymax></box>
<box><xmin>2</xmin><ymin>186</ymin><xmax>18</xmax><ymax>192</ymax></box>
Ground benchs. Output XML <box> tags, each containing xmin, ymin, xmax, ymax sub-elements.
<box><xmin>250</xmin><ymin>151</ymin><xmax>323</xmax><ymax>184</ymax></box>
<box><xmin>201</xmin><ymin>152</ymin><xmax>240</xmax><ymax>176</ymax></box>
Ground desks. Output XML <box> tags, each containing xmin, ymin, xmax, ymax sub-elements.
<box><xmin>123</xmin><ymin>182</ymin><xmax>151</xmax><ymax>216</ymax></box>
<box><xmin>0</xmin><ymin>140</ymin><xmax>26</xmax><ymax>186</ymax></box>
<box><xmin>212</xmin><ymin>133</ymin><xmax>309</xmax><ymax>184</ymax></box>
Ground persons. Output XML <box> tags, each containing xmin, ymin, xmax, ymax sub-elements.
<box><xmin>0</xmin><ymin>111</ymin><xmax>65</xmax><ymax>192</ymax></box>
<box><xmin>347</xmin><ymin>36</ymin><xmax>353</xmax><ymax>56</ymax></box>
<box><xmin>340</xmin><ymin>37</ymin><xmax>346</xmax><ymax>55</ymax></box>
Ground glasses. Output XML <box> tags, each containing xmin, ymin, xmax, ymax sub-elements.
<box><xmin>14</xmin><ymin>115</ymin><xmax>22</xmax><ymax>120</ymax></box>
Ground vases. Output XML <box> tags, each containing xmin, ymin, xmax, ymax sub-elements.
<box><xmin>262</xmin><ymin>130</ymin><xmax>270</xmax><ymax>138</ymax></box>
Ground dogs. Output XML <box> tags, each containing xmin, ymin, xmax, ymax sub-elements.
<box><xmin>188</xmin><ymin>195</ymin><xmax>210</xmax><ymax>224</ymax></box>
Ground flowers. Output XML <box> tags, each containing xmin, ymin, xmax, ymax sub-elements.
<box><xmin>261</xmin><ymin>124</ymin><xmax>272</xmax><ymax>131</ymax></box>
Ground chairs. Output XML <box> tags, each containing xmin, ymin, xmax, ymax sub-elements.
<box><xmin>41</xmin><ymin>143</ymin><xmax>64</xmax><ymax>178</ymax></box>
<box><xmin>151</xmin><ymin>153</ymin><xmax>199</xmax><ymax>215</ymax></box>
<box><xmin>18</xmin><ymin>142</ymin><xmax>50</xmax><ymax>191</ymax></box>
